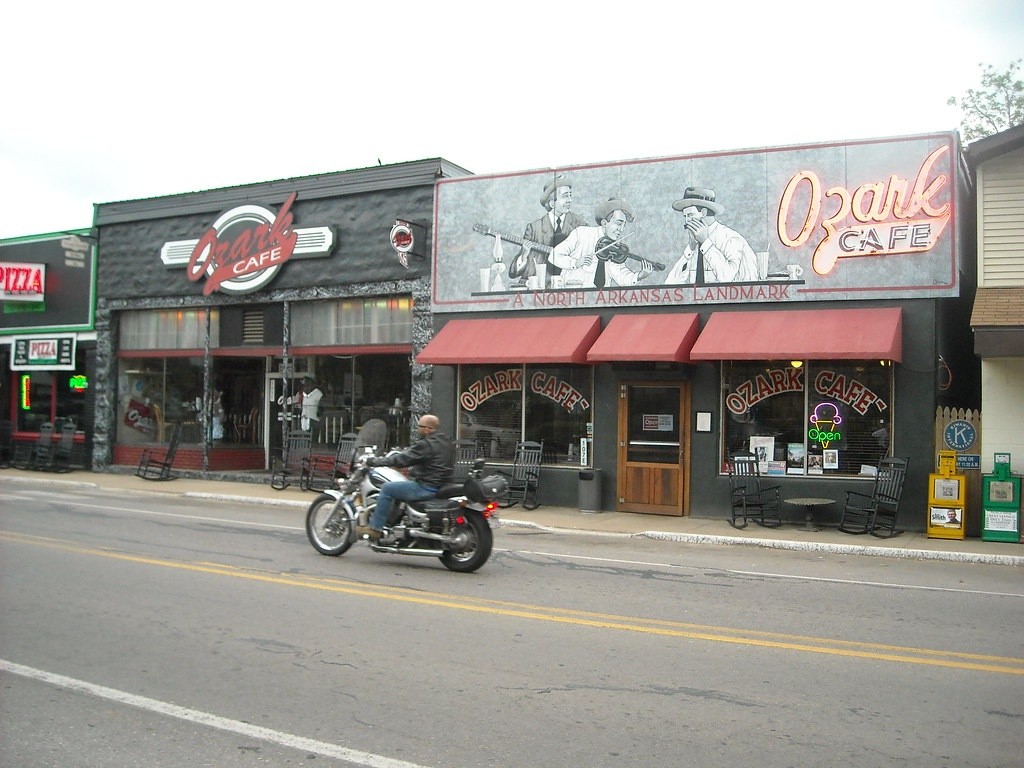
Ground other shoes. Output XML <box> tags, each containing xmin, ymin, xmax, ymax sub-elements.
<box><xmin>355</xmin><ymin>525</ymin><xmax>382</xmax><ymax>538</ymax></box>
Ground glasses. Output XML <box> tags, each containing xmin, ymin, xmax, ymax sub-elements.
<box><xmin>418</xmin><ymin>424</ymin><xmax>432</xmax><ymax>430</ymax></box>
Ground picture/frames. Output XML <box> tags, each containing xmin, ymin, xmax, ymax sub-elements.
<box><xmin>787</xmin><ymin>442</ymin><xmax>804</xmax><ymax>473</ymax></box>
<box><xmin>807</xmin><ymin>454</ymin><xmax>824</xmax><ymax>476</ymax></box>
<box><xmin>748</xmin><ymin>434</ymin><xmax>776</xmax><ymax>473</ymax></box>
<box><xmin>768</xmin><ymin>460</ymin><xmax>786</xmax><ymax>476</ymax></box>
<box><xmin>734</xmin><ymin>455</ymin><xmax>759</xmax><ymax>474</ymax></box>
<box><xmin>823</xmin><ymin>449</ymin><xmax>840</xmax><ymax>470</ymax></box>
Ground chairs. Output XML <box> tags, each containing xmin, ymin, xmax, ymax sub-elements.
<box><xmin>494</xmin><ymin>438</ymin><xmax>544</xmax><ymax>510</ymax></box>
<box><xmin>36</xmin><ymin>421</ymin><xmax>76</xmax><ymax>472</ymax></box>
<box><xmin>836</xmin><ymin>454</ymin><xmax>908</xmax><ymax>538</ymax></box>
<box><xmin>10</xmin><ymin>422</ymin><xmax>55</xmax><ymax>471</ymax></box>
<box><xmin>134</xmin><ymin>423</ymin><xmax>184</xmax><ymax>481</ymax></box>
<box><xmin>307</xmin><ymin>433</ymin><xmax>358</xmax><ymax>494</ymax></box>
<box><xmin>238</xmin><ymin>406</ymin><xmax>258</xmax><ymax>447</ymax></box>
<box><xmin>270</xmin><ymin>427</ymin><xmax>313</xmax><ymax>492</ymax></box>
<box><xmin>450</xmin><ymin>436</ymin><xmax>478</xmax><ymax>484</ymax></box>
<box><xmin>726</xmin><ymin>447</ymin><xmax>784</xmax><ymax>531</ymax></box>
<box><xmin>152</xmin><ymin>404</ymin><xmax>174</xmax><ymax>444</ymax></box>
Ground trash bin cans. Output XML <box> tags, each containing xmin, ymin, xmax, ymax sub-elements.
<box><xmin>577</xmin><ymin>468</ymin><xmax>604</xmax><ymax>515</ymax></box>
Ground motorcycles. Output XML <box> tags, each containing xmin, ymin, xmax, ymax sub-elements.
<box><xmin>306</xmin><ymin>417</ymin><xmax>509</xmax><ymax>572</ymax></box>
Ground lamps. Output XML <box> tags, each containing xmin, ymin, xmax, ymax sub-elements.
<box><xmin>880</xmin><ymin>359</ymin><xmax>892</xmax><ymax>368</ymax></box>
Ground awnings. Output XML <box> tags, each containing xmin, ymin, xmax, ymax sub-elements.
<box><xmin>690</xmin><ymin>307</ymin><xmax>903</xmax><ymax>364</ymax></box>
<box><xmin>587</xmin><ymin>313</ymin><xmax>699</xmax><ymax>367</ymax></box>
<box><xmin>416</xmin><ymin>315</ymin><xmax>601</xmax><ymax>365</ymax></box>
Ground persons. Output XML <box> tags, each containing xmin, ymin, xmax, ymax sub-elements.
<box><xmin>810</xmin><ymin>457</ymin><xmax>820</xmax><ymax>468</ymax></box>
<box><xmin>354</xmin><ymin>414</ymin><xmax>456</xmax><ymax>540</ymax></box>
<box><xmin>211</xmin><ymin>389</ymin><xmax>227</xmax><ymax>444</ymax></box>
<box><xmin>298</xmin><ymin>372</ymin><xmax>325</xmax><ymax>444</ymax></box>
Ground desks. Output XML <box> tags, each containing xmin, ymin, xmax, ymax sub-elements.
<box><xmin>783</xmin><ymin>499</ymin><xmax>836</xmax><ymax>532</ymax></box>
